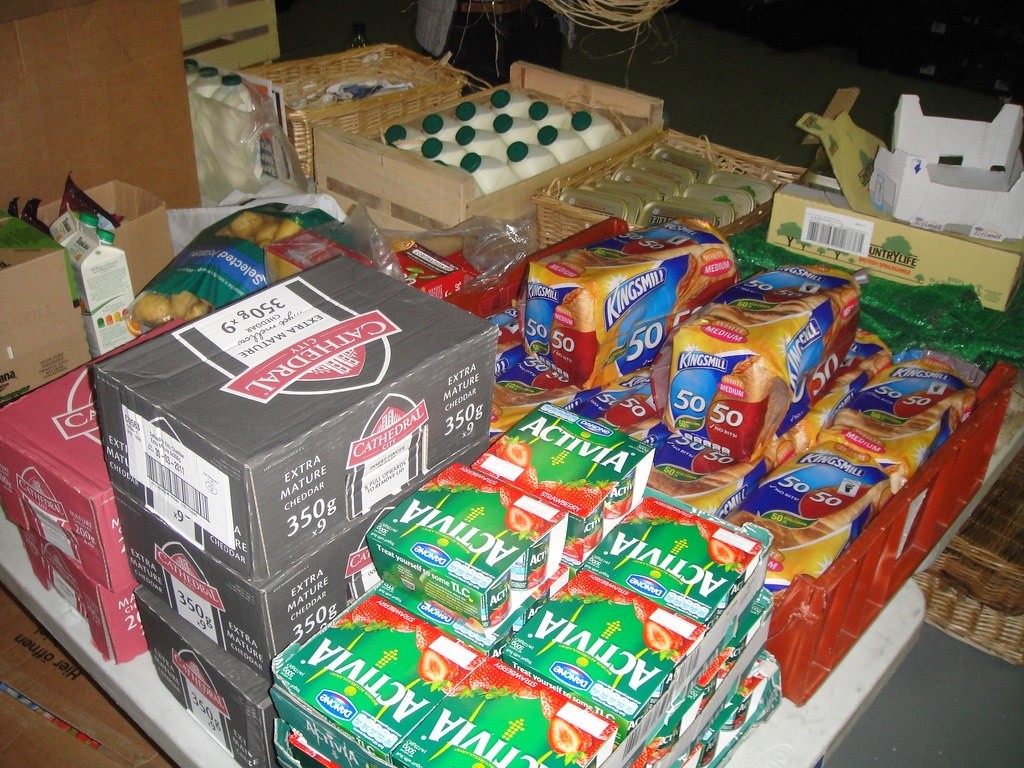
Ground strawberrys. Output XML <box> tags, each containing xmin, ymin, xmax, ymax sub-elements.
<box><xmin>349</xmin><ymin>430</ymin><xmax>763</xmax><ymax>767</ymax></box>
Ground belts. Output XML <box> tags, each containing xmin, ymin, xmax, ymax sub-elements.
<box><xmin>453</xmin><ymin>0</ymin><xmax>533</xmax><ymax>14</ymax></box>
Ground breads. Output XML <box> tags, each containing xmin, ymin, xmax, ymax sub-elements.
<box><xmin>486</xmin><ymin>218</ymin><xmax>972</xmax><ymax>552</ymax></box>
<box><xmin>134</xmin><ymin>214</ymin><xmax>302</xmax><ymax>324</ymax></box>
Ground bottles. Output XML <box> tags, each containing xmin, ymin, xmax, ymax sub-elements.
<box><xmin>385</xmin><ymin>88</ymin><xmax>620</xmax><ymax>195</ymax></box>
<box><xmin>181</xmin><ymin>58</ymin><xmax>264</xmax><ymax>188</ymax></box>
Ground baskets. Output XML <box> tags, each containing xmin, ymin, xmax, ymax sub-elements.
<box><xmin>530</xmin><ymin>129</ymin><xmax>807</xmax><ymax>253</ymax></box>
<box><xmin>236</xmin><ymin>43</ymin><xmax>468</xmax><ymax>179</ymax></box>
<box><xmin>913</xmin><ymin>447</ymin><xmax>1024</xmax><ymax>666</ymax></box>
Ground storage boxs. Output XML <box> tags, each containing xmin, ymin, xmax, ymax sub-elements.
<box><xmin>0</xmin><ymin>0</ymin><xmax>1024</xmax><ymax>768</ymax></box>
